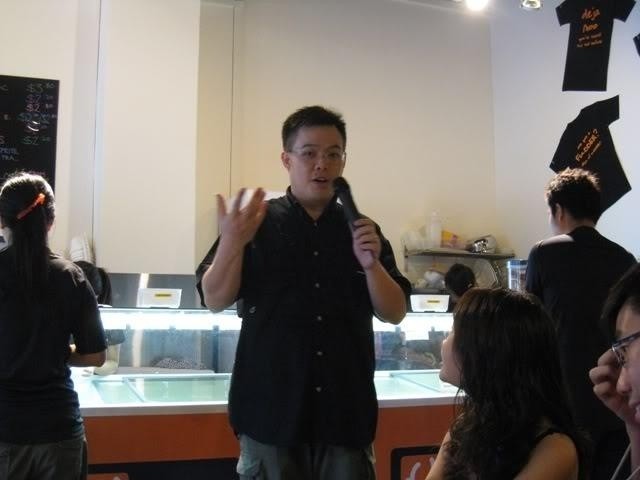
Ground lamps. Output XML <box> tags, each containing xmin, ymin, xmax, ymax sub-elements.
<box><xmin>518</xmin><ymin>0</ymin><xmax>544</xmax><ymax>13</ymax></box>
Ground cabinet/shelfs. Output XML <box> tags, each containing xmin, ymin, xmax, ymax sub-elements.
<box><xmin>402</xmin><ymin>245</ymin><xmax>517</xmax><ymax>295</ymax></box>
<box><xmin>67</xmin><ymin>306</ymin><xmax>242</xmax><ymax>464</ymax></box>
<box><xmin>370</xmin><ymin>310</ymin><xmax>466</xmax><ymax>479</ymax></box>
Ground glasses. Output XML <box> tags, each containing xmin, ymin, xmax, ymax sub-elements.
<box><xmin>288</xmin><ymin>147</ymin><xmax>350</xmax><ymax>164</ymax></box>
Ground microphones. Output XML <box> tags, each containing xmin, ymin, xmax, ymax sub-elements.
<box><xmin>332</xmin><ymin>176</ymin><xmax>363</xmax><ymax>231</ymax></box>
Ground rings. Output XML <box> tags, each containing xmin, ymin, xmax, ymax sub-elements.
<box><xmin>256</xmin><ymin>212</ymin><xmax>263</xmax><ymax>218</ymax></box>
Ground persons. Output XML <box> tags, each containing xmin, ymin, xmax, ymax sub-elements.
<box><xmin>423</xmin><ymin>286</ymin><xmax>584</xmax><ymax>480</ymax></box>
<box><xmin>70</xmin><ymin>260</ymin><xmax>127</xmax><ymax>377</ymax></box>
<box><xmin>393</xmin><ymin>331</ymin><xmax>444</xmax><ymax>373</ymax></box>
<box><xmin>444</xmin><ymin>263</ymin><xmax>476</xmax><ymax>313</ymax></box>
<box><xmin>587</xmin><ymin>262</ymin><xmax>640</xmax><ymax>480</ymax></box>
<box><xmin>194</xmin><ymin>105</ymin><xmax>412</xmax><ymax>480</ymax></box>
<box><xmin>0</xmin><ymin>172</ymin><xmax>109</xmax><ymax>480</ymax></box>
<box><xmin>525</xmin><ymin>169</ymin><xmax>638</xmax><ymax>480</ymax></box>
